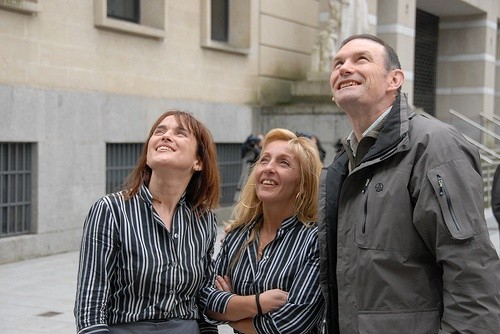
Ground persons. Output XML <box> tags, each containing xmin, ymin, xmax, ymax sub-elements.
<box><xmin>310</xmin><ymin>135</ymin><xmax>326</xmax><ymax>160</ymax></box>
<box><xmin>254</xmin><ymin>135</ymin><xmax>264</xmax><ymax>156</ymax></box>
<box><xmin>318</xmin><ymin>34</ymin><xmax>499</xmax><ymax>334</ymax></box>
<box><xmin>74</xmin><ymin>112</ymin><xmax>219</xmax><ymax>334</ymax></box>
<box><xmin>491</xmin><ymin>164</ymin><xmax>500</xmax><ymax>223</ymax></box>
<box><xmin>200</xmin><ymin>129</ymin><xmax>326</xmax><ymax>334</ymax></box>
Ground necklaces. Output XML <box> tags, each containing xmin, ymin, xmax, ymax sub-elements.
<box><xmin>258</xmin><ymin>230</ymin><xmax>262</xmax><ymax>256</ymax></box>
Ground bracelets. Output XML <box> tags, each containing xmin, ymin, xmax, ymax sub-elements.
<box><xmin>256</xmin><ymin>293</ymin><xmax>263</xmax><ymax>314</ymax></box>
